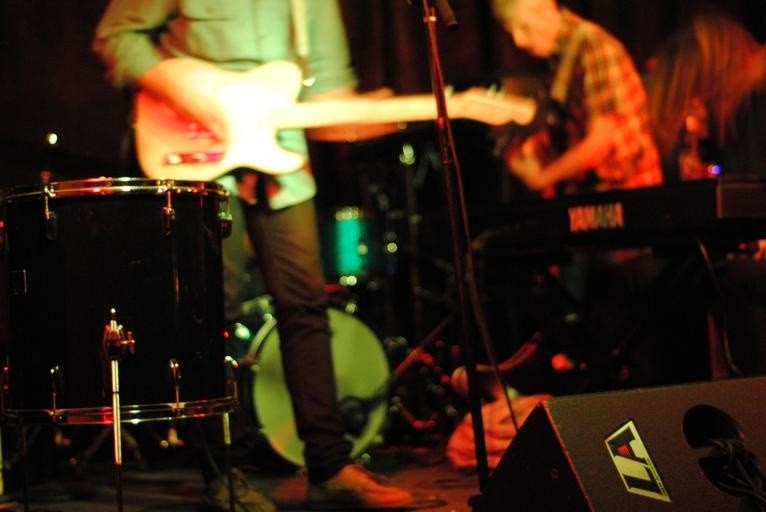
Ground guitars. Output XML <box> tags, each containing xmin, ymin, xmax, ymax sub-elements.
<box><xmin>501</xmin><ymin>21</ymin><xmax>594</xmax><ymax>199</ymax></box>
<box><xmin>134</xmin><ymin>58</ymin><xmax>537</xmax><ymax>182</ymax></box>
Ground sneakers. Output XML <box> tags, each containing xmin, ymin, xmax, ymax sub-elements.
<box><xmin>306</xmin><ymin>464</ymin><xmax>412</xmax><ymax>508</ymax></box>
<box><xmin>210</xmin><ymin>473</ymin><xmax>275</xmax><ymax>512</ymax></box>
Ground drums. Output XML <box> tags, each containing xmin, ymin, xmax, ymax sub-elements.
<box><xmin>236</xmin><ymin>296</ymin><xmax>391</xmax><ymax>470</ymax></box>
<box><xmin>3</xmin><ymin>178</ymin><xmax>237</xmax><ymax>427</ymax></box>
<box><xmin>324</xmin><ymin>206</ymin><xmax>378</xmax><ymax>284</ymax></box>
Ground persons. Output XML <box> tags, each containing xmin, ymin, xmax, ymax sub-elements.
<box><xmin>649</xmin><ymin>14</ymin><xmax>765</xmax><ymax>174</ymax></box>
<box><xmin>493</xmin><ymin>1</ymin><xmax>664</xmax><ymax>199</ymax></box>
<box><xmin>92</xmin><ymin>0</ymin><xmax>412</xmax><ymax>512</ymax></box>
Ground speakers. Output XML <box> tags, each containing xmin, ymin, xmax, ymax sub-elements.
<box><xmin>481</xmin><ymin>376</ymin><xmax>766</xmax><ymax>512</ymax></box>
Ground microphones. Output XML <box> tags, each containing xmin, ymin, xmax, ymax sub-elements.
<box><xmin>433</xmin><ymin>0</ymin><xmax>459</xmax><ymax>29</ymax></box>
